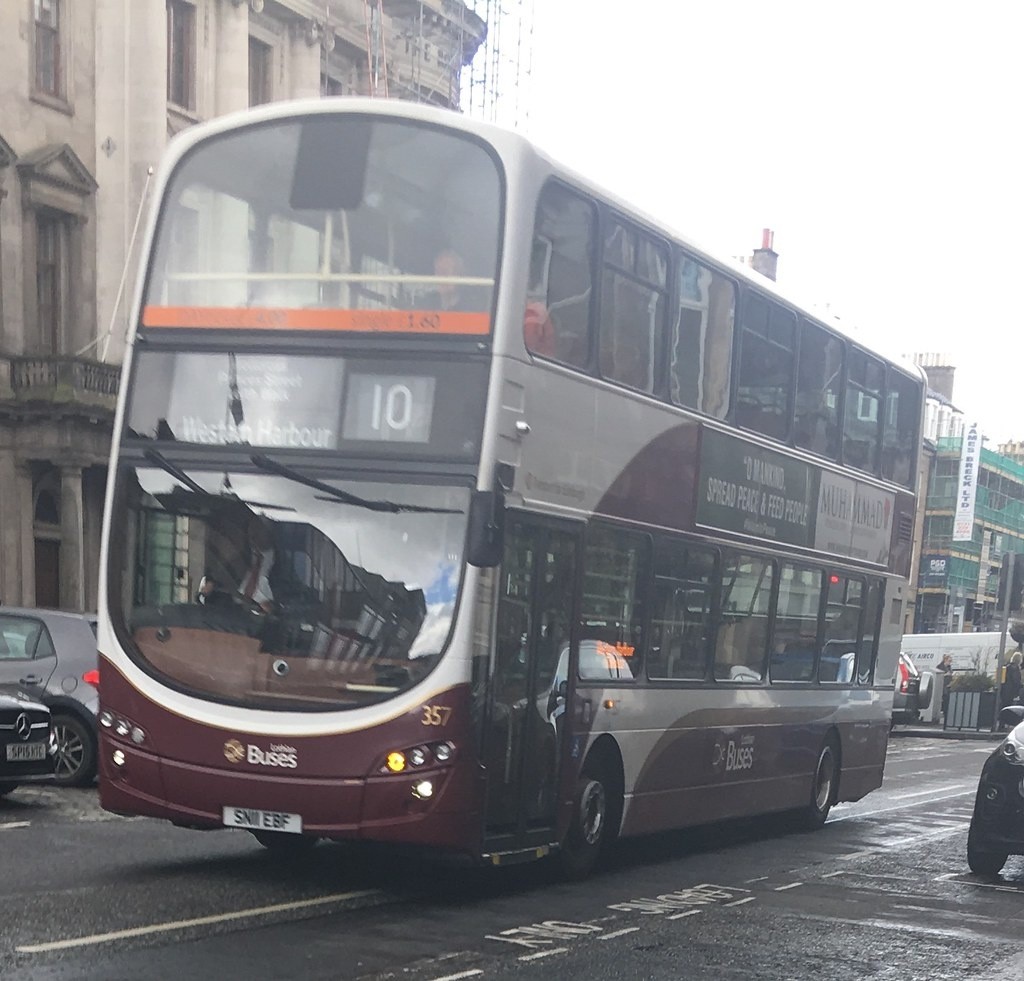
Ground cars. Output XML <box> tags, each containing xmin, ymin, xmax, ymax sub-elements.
<box><xmin>493</xmin><ymin>641</ymin><xmax>633</xmax><ymax>727</ymax></box>
<box><xmin>1</xmin><ymin>683</ymin><xmax>55</xmax><ymax>797</ymax></box>
<box><xmin>646</xmin><ymin>660</ymin><xmax>763</xmax><ymax>682</ymax></box>
<box><xmin>966</xmin><ymin>704</ymin><xmax>1024</xmax><ymax>878</ymax></box>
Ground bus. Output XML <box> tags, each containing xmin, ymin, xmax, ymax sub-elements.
<box><xmin>95</xmin><ymin>95</ymin><xmax>928</xmax><ymax>884</ymax></box>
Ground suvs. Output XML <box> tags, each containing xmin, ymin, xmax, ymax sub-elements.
<box><xmin>890</xmin><ymin>650</ymin><xmax>933</xmax><ymax>728</ymax></box>
<box><xmin>0</xmin><ymin>604</ymin><xmax>104</xmax><ymax>787</ymax></box>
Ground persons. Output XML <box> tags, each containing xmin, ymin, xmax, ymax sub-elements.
<box><xmin>938</xmin><ymin>653</ymin><xmax>954</xmax><ymax>714</ymax></box>
<box><xmin>417</xmin><ymin>255</ymin><xmax>485</xmax><ymax>311</ymax></box>
<box><xmin>999</xmin><ymin>651</ymin><xmax>1022</xmax><ymax>729</ymax></box>
<box><xmin>196</xmin><ymin>516</ymin><xmax>300</xmax><ymax>614</ymax></box>
